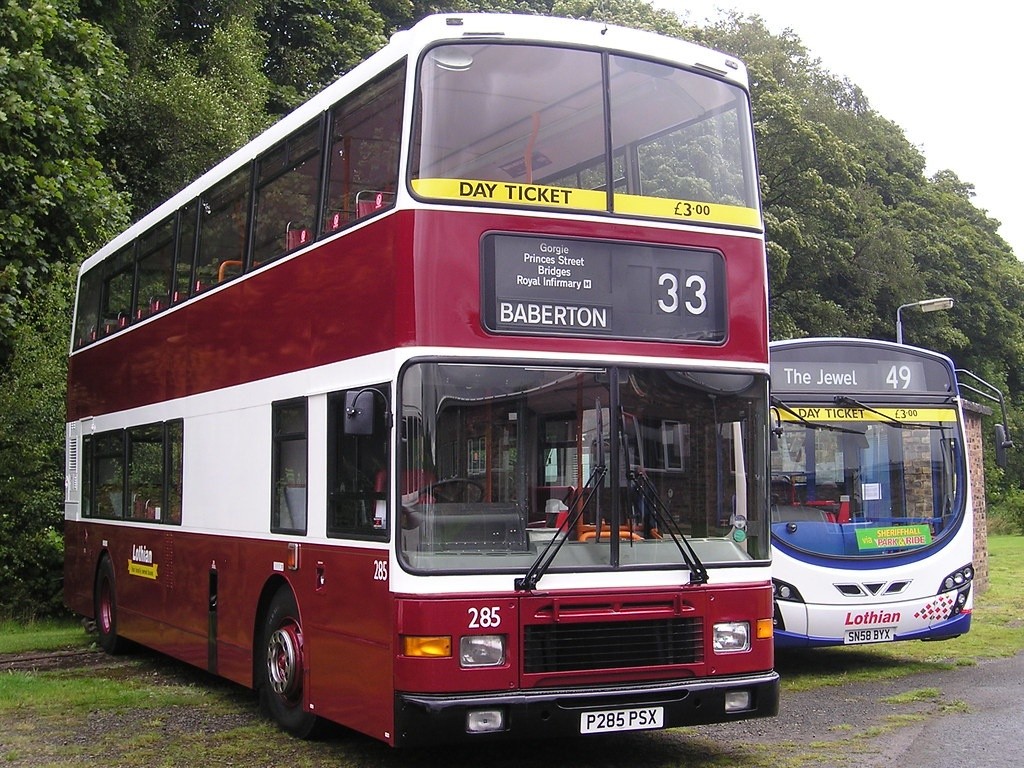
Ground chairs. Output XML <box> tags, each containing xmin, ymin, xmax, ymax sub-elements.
<box><xmin>373</xmin><ymin>464</ymin><xmax>435</xmax><ymax>527</ymax></box>
<box><xmin>95</xmin><ymin>485</ymin><xmax>180</xmax><ymax>525</ymax></box>
<box><xmin>769</xmin><ymin>471</ymin><xmax>842</xmax><ymax>510</ymax></box>
<box><xmin>74</xmin><ymin>188</ymin><xmax>397</xmax><ymax>350</ymax></box>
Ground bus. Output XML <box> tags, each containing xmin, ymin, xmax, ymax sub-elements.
<box><xmin>59</xmin><ymin>9</ymin><xmax>782</xmax><ymax>753</ymax></box>
<box><xmin>588</xmin><ymin>335</ymin><xmax>1014</xmax><ymax>661</ymax></box>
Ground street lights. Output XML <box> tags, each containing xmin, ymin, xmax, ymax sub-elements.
<box><xmin>894</xmin><ymin>298</ymin><xmax>955</xmax><ymax>345</ymax></box>
